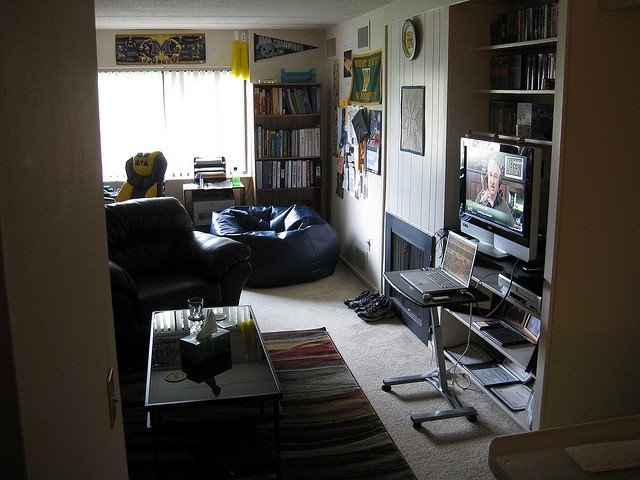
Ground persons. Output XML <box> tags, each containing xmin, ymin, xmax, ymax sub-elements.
<box><xmin>475</xmin><ymin>159</ymin><xmax>514</xmax><ymax>227</ymax></box>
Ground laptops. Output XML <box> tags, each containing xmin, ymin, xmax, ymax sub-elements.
<box><xmin>399</xmin><ymin>230</ymin><xmax>479</xmax><ymax>296</ymax></box>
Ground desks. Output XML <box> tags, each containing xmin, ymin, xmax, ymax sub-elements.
<box><xmin>382</xmin><ymin>266</ymin><xmax>491</xmax><ymax>428</ymax></box>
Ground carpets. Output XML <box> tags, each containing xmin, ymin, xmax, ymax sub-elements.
<box><xmin>116</xmin><ymin>325</ymin><xmax>421</xmax><ymax>479</ymax></box>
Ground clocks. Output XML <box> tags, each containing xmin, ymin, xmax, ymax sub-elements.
<box><xmin>401</xmin><ymin>19</ymin><xmax>419</xmax><ymax>60</ymax></box>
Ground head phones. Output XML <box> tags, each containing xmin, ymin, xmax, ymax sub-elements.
<box><xmin>484</xmin><ymin>158</ymin><xmax>503</xmax><ymax>189</ymax></box>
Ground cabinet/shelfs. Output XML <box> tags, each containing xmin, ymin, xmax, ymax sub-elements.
<box><xmin>446</xmin><ymin>0</ymin><xmax>640</xmax><ymax>429</ymax></box>
<box><xmin>184</xmin><ymin>181</ymin><xmax>246</xmax><ymax>229</ymax></box>
<box><xmin>252</xmin><ymin>81</ymin><xmax>330</xmax><ymax>218</ymax></box>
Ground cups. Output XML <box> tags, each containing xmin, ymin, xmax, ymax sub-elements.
<box><xmin>187</xmin><ymin>297</ymin><xmax>205</xmax><ymax>320</ymax></box>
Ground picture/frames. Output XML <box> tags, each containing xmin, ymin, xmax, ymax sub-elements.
<box><xmin>400</xmin><ymin>85</ymin><xmax>425</xmax><ymax>157</ymax></box>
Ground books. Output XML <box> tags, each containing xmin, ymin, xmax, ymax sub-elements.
<box><xmin>489</xmin><ymin>53</ymin><xmax>556</xmax><ymax>90</ymax></box>
<box><xmin>254</xmin><ymin>86</ymin><xmax>320</xmax><ymax>115</ymax></box>
<box><xmin>256</xmin><ymin>124</ymin><xmax>320</xmax><ymax>158</ymax></box>
<box><xmin>256</xmin><ymin>159</ymin><xmax>321</xmax><ymax>189</ymax></box>
<box><xmin>488</xmin><ymin>100</ymin><xmax>551</xmax><ymax>138</ymax></box>
<box><xmin>490</xmin><ymin>3</ymin><xmax>559</xmax><ymax>45</ymax></box>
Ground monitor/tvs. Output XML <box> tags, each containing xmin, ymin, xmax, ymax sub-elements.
<box><xmin>458</xmin><ymin>136</ymin><xmax>542</xmax><ymax>283</ymax></box>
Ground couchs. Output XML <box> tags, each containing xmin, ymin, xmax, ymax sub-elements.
<box><xmin>104</xmin><ymin>194</ymin><xmax>254</xmax><ymax>320</ymax></box>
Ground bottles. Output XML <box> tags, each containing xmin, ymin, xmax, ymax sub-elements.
<box><xmin>231</xmin><ymin>167</ymin><xmax>240</xmax><ymax>186</ymax></box>
<box><xmin>199</xmin><ymin>175</ymin><xmax>204</xmax><ymax>188</ymax></box>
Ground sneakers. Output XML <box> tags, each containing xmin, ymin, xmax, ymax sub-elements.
<box><xmin>354</xmin><ymin>294</ymin><xmax>387</xmax><ymax>313</ymax></box>
<box><xmin>349</xmin><ymin>289</ymin><xmax>380</xmax><ymax>309</ymax></box>
<box><xmin>357</xmin><ymin>299</ymin><xmax>395</xmax><ymax>322</ymax></box>
<box><xmin>344</xmin><ymin>288</ymin><xmax>371</xmax><ymax>305</ymax></box>
